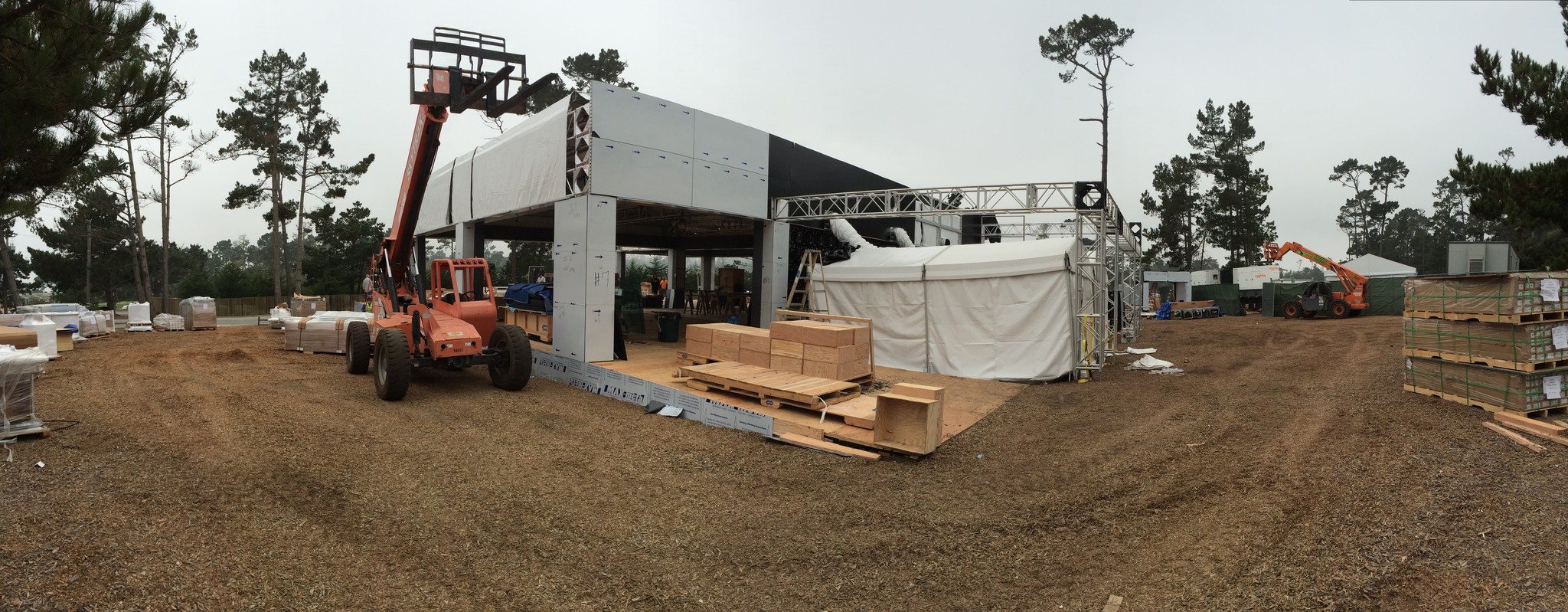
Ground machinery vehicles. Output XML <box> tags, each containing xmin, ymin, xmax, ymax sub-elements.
<box><xmin>344</xmin><ymin>26</ymin><xmax>558</xmax><ymax>402</ymax></box>
<box><xmin>1263</xmin><ymin>241</ymin><xmax>1370</xmax><ymax>318</ymax></box>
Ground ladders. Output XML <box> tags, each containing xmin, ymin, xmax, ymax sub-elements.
<box><xmin>1141</xmin><ymin>289</ymin><xmax>1157</xmax><ymax>310</ymax></box>
<box><xmin>1167</xmin><ymin>289</ymin><xmax>1176</xmax><ymax>303</ymax></box>
<box><xmin>783</xmin><ymin>249</ymin><xmax>829</xmax><ymax>321</ymax></box>
<box><xmin>1149</xmin><ymin>283</ymin><xmax>1161</xmax><ymax>311</ymax></box>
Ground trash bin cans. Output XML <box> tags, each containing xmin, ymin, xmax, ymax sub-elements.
<box><xmin>655</xmin><ymin>312</ymin><xmax>680</xmax><ymax>343</ymax></box>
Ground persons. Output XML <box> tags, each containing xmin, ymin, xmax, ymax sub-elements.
<box><xmin>536</xmin><ymin>273</ymin><xmax>546</xmax><ymax>284</ymax></box>
<box><xmin>361</xmin><ymin>296</ymin><xmax>373</xmax><ymax>313</ymax></box>
<box><xmin>362</xmin><ymin>274</ymin><xmax>373</xmax><ymax>301</ymax></box>
<box><xmin>651</xmin><ymin>273</ymin><xmax>667</xmax><ymax>300</ymax></box>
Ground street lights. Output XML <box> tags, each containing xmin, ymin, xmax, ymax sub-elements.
<box><xmin>215</xmin><ymin>267</ymin><xmax>219</xmax><ymax>276</ymax></box>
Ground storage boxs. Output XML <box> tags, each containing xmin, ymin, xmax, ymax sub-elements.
<box><xmin>685</xmin><ymin>314</ymin><xmax>947</xmax><ymax>458</ymax></box>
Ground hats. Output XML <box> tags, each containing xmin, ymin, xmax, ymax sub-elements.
<box><xmin>367</xmin><ymin>296</ymin><xmax>372</xmax><ymax>299</ymax></box>
<box><xmin>537</xmin><ymin>273</ymin><xmax>542</xmax><ymax>276</ymax></box>
